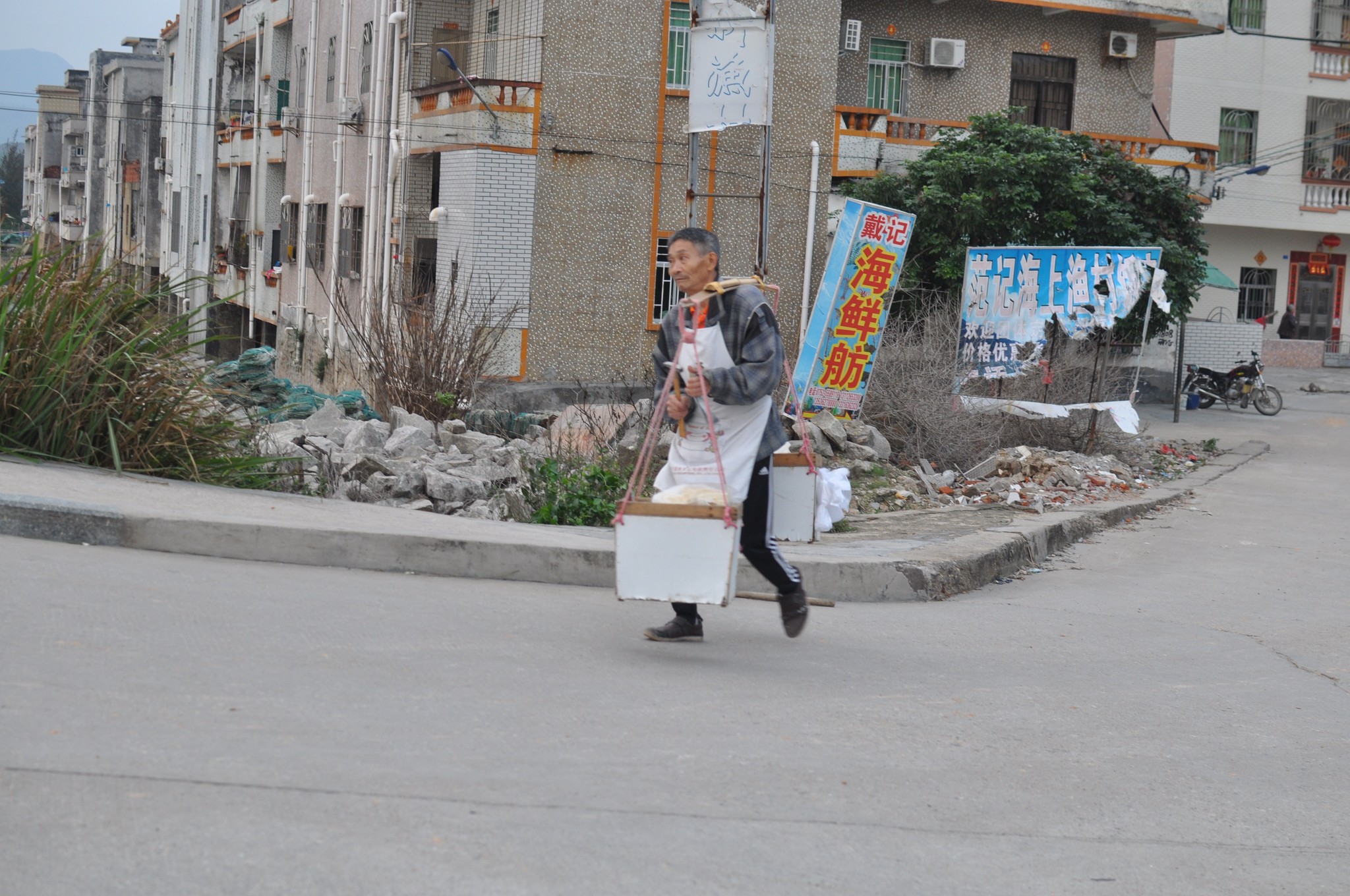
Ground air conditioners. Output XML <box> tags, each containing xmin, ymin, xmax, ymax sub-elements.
<box><xmin>925</xmin><ymin>38</ymin><xmax>966</xmax><ymax>68</ymax></box>
<box><xmin>1107</xmin><ymin>31</ymin><xmax>1138</xmax><ymax>59</ymax></box>
<box><xmin>154</xmin><ymin>158</ymin><xmax>163</xmax><ymax>171</ymax></box>
<box><xmin>840</xmin><ymin>19</ymin><xmax>862</xmax><ymax>51</ymax></box>
<box><xmin>341</xmin><ymin>97</ymin><xmax>360</xmax><ymax>123</ymax></box>
<box><xmin>280</xmin><ymin>107</ymin><xmax>297</xmax><ymax>128</ymax></box>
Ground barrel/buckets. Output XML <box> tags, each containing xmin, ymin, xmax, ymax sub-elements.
<box><xmin>1181</xmin><ymin>390</ymin><xmax>1200</xmax><ymax>410</ymax></box>
<box><xmin>1173</xmin><ymin>394</ymin><xmax>1188</xmax><ymax>411</ymax></box>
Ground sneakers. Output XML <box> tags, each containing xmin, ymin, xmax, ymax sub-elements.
<box><xmin>644</xmin><ymin>611</ymin><xmax>704</xmax><ymax>641</ymax></box>
<box><xmin>776</xmin><ymin>590</ymin><xmax>806</xmax><ymax>638</ymax></box>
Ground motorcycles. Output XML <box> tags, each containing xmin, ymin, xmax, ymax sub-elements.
<box><xmin>1181</xmin><ymin>350</ymin><xmax>1282</xmax><ymax>416</ymax></box>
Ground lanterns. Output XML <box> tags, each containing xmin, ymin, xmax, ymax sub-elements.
<box><xmin>1323</xmin><ymin>233</ymin><xmax>1342</xmax><ymax>251</ymax></box>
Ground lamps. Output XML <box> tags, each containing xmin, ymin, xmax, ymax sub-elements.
<box><xmin>1212</xmin><ymin>166</ymin><xmax>1271</xmax><ymax>199</ymax></box>
<box><xmin>437</xmin><ymin>48</ymin><xmax>500</xmax><ymax>139</ymax></box>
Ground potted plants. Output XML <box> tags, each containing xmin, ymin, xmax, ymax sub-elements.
<box><xmin>1317</xmin><ymin>157</ymin><xmax>1330</xmax><ymax>177</ymax></box>
<box><xmin>217</xmin><ymin>117</ymin><xmax>225</xmax><ymax>130</ymax></box>
<box><xmin>230</xmin><ymin>115</ymin><xmax>241</xmax><ymax>127</ymax></box>
<box><xmin>49</xmin><ymin>211</ymin><xmax>59</xmax><ymax>222</ymax></box>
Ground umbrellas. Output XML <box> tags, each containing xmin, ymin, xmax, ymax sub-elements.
<box><xmin>1196</xmin><ymin>258</ymin><xmax>1240</xmax><ymax>293</ymax></box>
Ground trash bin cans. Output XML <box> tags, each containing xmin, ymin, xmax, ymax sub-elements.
<box><xmin>1184</xmin><ymin>394</ymin><xmax>1200</xmax><ymax>410</ymax></box>
<box><xmin>1174</xmin><ymin>394</ymin><xmax>1188</xmax><ymax>410</ymax></box>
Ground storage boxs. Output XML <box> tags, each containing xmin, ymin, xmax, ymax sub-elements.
<box><xmin>614</xmin><ymin>498</ymin><xmax>742</xmax><ymax>606</ymax></box>
<box><xmin>774</xmin><ymin>453</ymin><xmax>822</xmax><ymax>542</ymax></box>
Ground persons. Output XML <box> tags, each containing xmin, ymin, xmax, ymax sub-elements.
<box><xmin>643</xmin><ymin>228</ymin><xmax>808</xmax><ymax>642</ymax></box>
<box><xmin>1277</xmin><ymin>304</ymin><xmax>1298</xmax><ymax>339</ymax></box>
